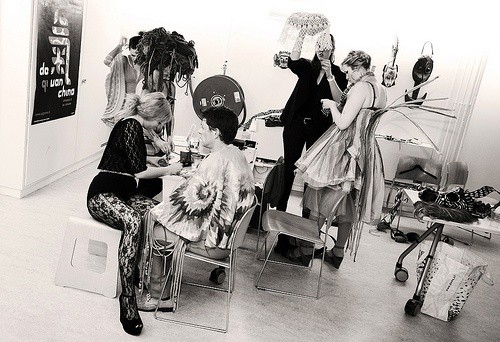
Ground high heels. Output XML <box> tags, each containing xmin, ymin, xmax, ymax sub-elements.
<box><xmin>119</xmin><ymin>294</ymin><xmax>144</xmax><ymax>337</ymax></box>
<box><xmin>321</xmin><ymin>246</ymin><xmax>344</xmax><ymax>269</ymax></box>
<box><xmin>288</xmin><ymin>245</ymin><xmax>314</xmax><ymax>267</ymax></box>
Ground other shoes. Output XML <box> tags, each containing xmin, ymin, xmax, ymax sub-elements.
<box><xmin>390</xmin><ymin>228</ymin><xmax>420</xmax><ymax>243</ymax></box>
<box><xmin>134</xmin><ymin>297</ymin><xmax>174</xmax><ymax>313</ymax></box>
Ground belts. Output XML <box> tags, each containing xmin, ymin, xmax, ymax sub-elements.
<box><xmin>301</xmin><ymin>117</ymin><xmax>311</xmax><ymax>125</ymax></box>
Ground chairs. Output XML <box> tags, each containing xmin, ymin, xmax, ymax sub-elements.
<box><xmin>153</xmin><ymin>148</ymin><xmax>348</xmax><ymax>334</ymax></box>
<box><xmin>384</xmin><ymin>156</ymin><xmax>491</xmax><ymax>245</ymax></box>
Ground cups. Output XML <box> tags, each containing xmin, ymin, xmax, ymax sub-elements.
<box><xmin>191</xmin><ymin>154</ymin><xmax>202</xmax><ymax>169</ymax></box>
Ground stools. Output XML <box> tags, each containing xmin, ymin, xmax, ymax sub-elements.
<box><xmin>53</xmin><ymin>211</ymin><xmax>125</xmax><ymax>299</ymax></box>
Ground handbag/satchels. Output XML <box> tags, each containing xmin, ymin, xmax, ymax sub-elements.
<box><xmin>275</xmin><ymin>230</ymin><xmax>337</xmax><ymax>256</ymax></box>
<box><xmin>416</xmin><ymin>240</ymin><xmax>494</xmax><ymax>322</ymax></box>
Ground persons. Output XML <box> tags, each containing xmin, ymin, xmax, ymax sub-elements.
<box><xmin>136</xmin><ymin>106</ymin><xmax>256</xmax><ymax>314</ymax></box>
<box><xmin>284</xmin><ymin>51</ymin><xmax>387</xmax><ymax>269</ymax></box>
<box><xmin>274</xmin><ymin>11</ymin><xmax>348</xmax><ymax>211</ymax></box>
<box><xmin>101</xmin><ymin>27</ymin><xmax>199</xmax><ymax>158</ymax></box>
<box><xmin>87</xmin><ymin>92</ymin><xmax>184</xmax><ymax>337</ymax></box>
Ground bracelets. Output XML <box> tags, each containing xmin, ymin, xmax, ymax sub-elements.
<box><xmin>327</xmin><ymin>75</ymin><xmax>335</xmax><ymax>82</ymax></box>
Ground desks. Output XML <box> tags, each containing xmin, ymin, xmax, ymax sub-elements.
<box><xmin>393</xmin><ymin>188</ymin><xmax>500</xmax><ymax>315</ymax></box>
